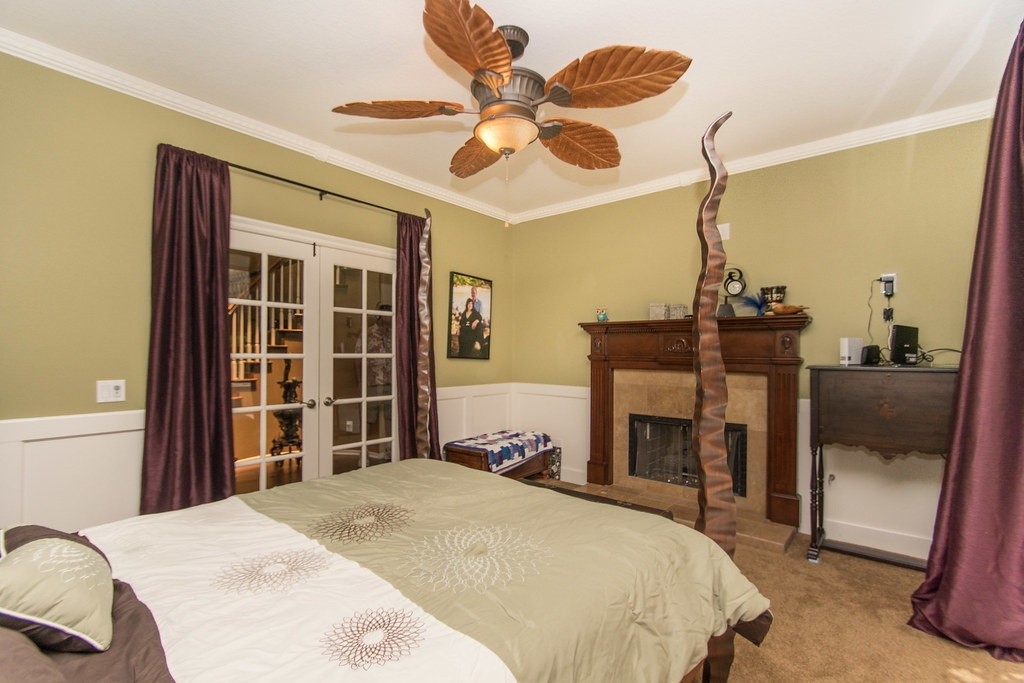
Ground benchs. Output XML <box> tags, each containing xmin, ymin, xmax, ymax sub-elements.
<box><xmin>441</xmin><ymin>429</ymin><xmax>551</xmax><ymax>481</ymax></box>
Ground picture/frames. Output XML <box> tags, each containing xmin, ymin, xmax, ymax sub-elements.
<box><xmin>446</xmin><ymin>270</ymin><xmax>493</xmax><ymax>360</ymax></box>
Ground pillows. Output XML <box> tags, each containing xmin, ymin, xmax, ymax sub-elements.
<box><xmin>0</xmin><ymin>524</ymin><xmax>115</xmax><ymax>655</ymax></box>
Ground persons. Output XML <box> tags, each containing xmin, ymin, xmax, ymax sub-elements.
<box><xmin>457</xmin><ymin>287</ymin><xmax>482</xmax><ymax>356</ymax></box>
<box><xmin>354</xmin><ymin>305</ymin><xmax>392</xmax><ymax>467</ymax></box>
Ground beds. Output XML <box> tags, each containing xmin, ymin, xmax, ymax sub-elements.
<box><xmin>0</xmin><ymin>455</ymin><xmax>774</xmax><ymax>683</ymax></box>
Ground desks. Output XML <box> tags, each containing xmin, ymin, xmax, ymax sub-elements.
<box><xmin>808</xmin><ymin>365</ymin><xmax>962</xmax><ymax>573</ymax></box>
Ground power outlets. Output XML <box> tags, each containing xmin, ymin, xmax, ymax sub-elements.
<box><xmin>881</xmin><ymin>272</ymin><xmax>897</xmax><ymax>293</ymax></box>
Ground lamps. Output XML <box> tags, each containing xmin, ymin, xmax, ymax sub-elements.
<box><xmin>474</xmin><ymin>114</ymin><xmax>539</xmax><ymax>231</ymax></box>
<box><xmin>330</xmin><ymin>1</ymin><xmax>693</xmax><ymax>181</ymax></box>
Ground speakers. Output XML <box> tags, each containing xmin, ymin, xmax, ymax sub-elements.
<box><xmin>891</xmin><ymin>325</ymin><xmax>920</xmax><ymax>365</ymax></box>
